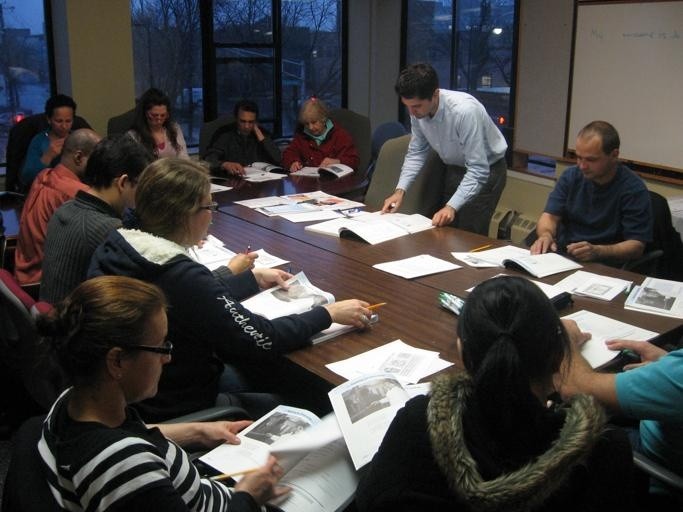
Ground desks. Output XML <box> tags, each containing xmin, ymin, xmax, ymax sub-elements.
<box><xmin>217</xmin><ymin>205</ymin><xmax>681</xmax><ymax>402</ymax></box>
<box><xmin>202</xmin><ymin>208</ymin><xmax>467</xmax><ymax>391</ymax></box>
<box><xmin>207</xmin><ymin>165</ymin><xmax>370</xmax><ymax>208</ymax></box>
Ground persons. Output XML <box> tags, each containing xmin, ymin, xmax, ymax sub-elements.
<box><xmin>529</xmin><ymin>119</ymin><xmax>653</xmax><ymax>269</ymax></box>
<box><xmin>551</xmin><ymin>317</ymin><xmax>681</xmax><ymax>510</ymax></box>
<box><xmin>381</xmin><ymin>62</ymin><xmax>510</xmax><ymax>237</ymax></box>
<box><xmin>12</xmin><ymin>85</ymin><xmax>357</xmax><ymax>310</ymax></box>
<box><xmin>88</xmin><ymin>154</ymin><xmax>374</xmax><ymax>419</ymax></box>
<box><xmin>34</xmin><ymin>273</ymin><xmax>294</xmax><ymax>511</ymax></box>
<box><xmin>356</xmin><ymin>274</ymin><xmax>636</xmax><ymax>510</ymax></box>
<box><xmin>184</xmin><ymin>157</ymin><xmax>682</xmax><ymax>511</ymax></box>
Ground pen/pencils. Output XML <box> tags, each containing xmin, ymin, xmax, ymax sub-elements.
<box><xmin>468</xmin><ymin>245</ymin><xmax>492</xmax><ymax>252</ymax></box>
<box><xmin>245</xmin><ymin>245</ymin><xmax>251</xmax><ymax>255</ymax></box>
<box><xmin>366</xmin><ymin>302</ymin><xmax>387</xmax><ymax>310</ymax></box>
<box><xmin>210</xmin><ymin>470</ymin><xmax>257</xmax><ymax>481</ymax></box>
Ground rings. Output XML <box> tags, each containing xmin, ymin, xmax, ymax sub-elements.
<box><xmin>360</xmin><ymin>313</ymin><xmax>370</xmax><ymax>326</ymax></box>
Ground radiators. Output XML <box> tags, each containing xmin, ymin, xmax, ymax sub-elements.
<box><xmin>489</xmin><ymin>206</ymin><xmax>538</xmax><ymax>245</ymax></box>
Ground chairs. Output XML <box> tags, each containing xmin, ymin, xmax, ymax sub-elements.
<box><xmin>6</xmin><ymin>111</ymin><xmax>91</xmax><ymax>191</ymax></box>
<box><xmin>107</xmin><ymin>110</ymin><xmax>141</xmax><ymax>145</ymax></box>
<box><xmin>324</xmin><ymin>109</ymin><xmax>370</xmax><ymax>176</ymax></box>
<box><xmin>366</xmin><ymin>134</ymin><xmax>423</xmax><ymax>213</ymax></box>
<box><xmin>1</xmin><ymin>268</ymin><xmax>30</xmax><ymax>320</ymax></box>
<box><xmin>633</xmin><ymin>449</ymin><xmax>682</xmax><ymax>512</ymax></box>
<box><xmin>15</xmin><ymin>405</ymin><xmax>255</xmax><ymax>512</ymax></box>
<box><xmin>199</xmin><ymin>115</ymin><xmax>232</xmax><ymax>160</ymax></box>
<box><xmin>30</xmin><ymin>302</ymin><xmax>59</xmax><ymax>324</ymax></box>
<box><xmin>619</xmin><ymin>190</ymin><xmax>679</xmax><ymax>279</ymax></box>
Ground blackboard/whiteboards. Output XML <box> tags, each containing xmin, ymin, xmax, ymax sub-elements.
<box><xmin>565</xmin><ymin>0</ymin><xmax>683</xmax><ymax>180</ymax></box>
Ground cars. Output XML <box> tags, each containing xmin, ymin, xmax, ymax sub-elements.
<box><xmin>456</xmin><ymin>84</ymin><xmax>513</xmax><ymax>131</ymax></box>
<box><xmin>0</xmin><ymin>105</ymin><xmax>40</xmax><ymax>131</ymax></box>
<box><xmin>170</xmin><ymin>82</ymin><xmax>204</xmax><ymax>121</ymax></box>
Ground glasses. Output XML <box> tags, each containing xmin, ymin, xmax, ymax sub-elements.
<box><xmin>134</xmin><ymin>341</ymin><xmax>173</xmax><ymax>354</ymax></box>
<box><xmin>148</xmin><ymin>114</ymin><xmax>170</xmax><ymax>122</ymax></box>
<box><xmin>199</xmin><ymin>202</ymin><xmax>218</xmax><ymax>212</ymax></box>
